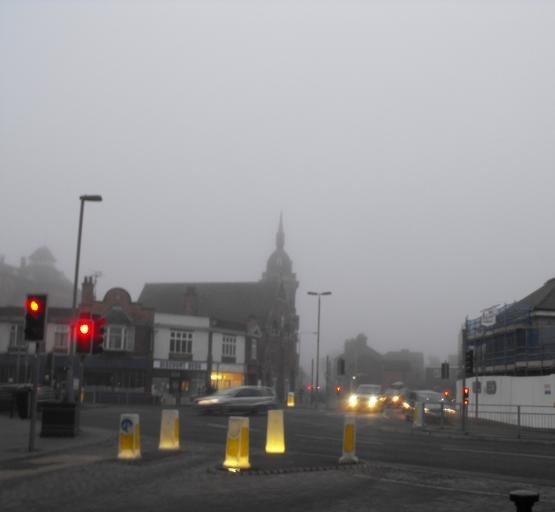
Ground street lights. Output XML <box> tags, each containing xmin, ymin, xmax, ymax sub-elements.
<box><xmin>306</xmin><ymin>289</ymin><xmax>332</xmax><ymax>404</ymax></box>
<box><xmin>69</xmin><ymin>193</ymin><xmax>103</xmax><ymax>323</ymax></box>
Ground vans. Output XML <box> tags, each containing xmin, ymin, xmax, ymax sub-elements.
<box><xmin>199</xmin><ymin>384</ymin><xmax>278</xmax><ymax>417</ymax></box>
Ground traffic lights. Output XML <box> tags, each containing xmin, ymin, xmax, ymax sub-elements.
<box><xmin>24</xmin><ymin>293</ymin><xmax>46</xmax><ymax>342</ymax></box>
<box><xmin>465</xmin><ymin>349</ymin><xmax>474</xmax><ymax>373</ymax></box>
<box><xmin>73</xmin><ymin>319</ymin><xmax>93</xmax><ymax>355</ymax></box>
<box><xmin>464</xmin><ymin>387</ymin><xmax>470</xmax><ymax>407</ymax></box>
<box><xmin>93</xmin><ymin>319</ymin><xmax>106</xmax><ymax>355</ymax></box>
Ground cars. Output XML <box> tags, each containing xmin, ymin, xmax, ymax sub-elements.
<box><xmin>403</xmin><ymin>389</ymin><xmax>456</xmax><ymax>424</ymax></box>
<box><xmin>351</xmin><ymin>381</ymin><xmax>408</xmax><ymax>413</ymax></box>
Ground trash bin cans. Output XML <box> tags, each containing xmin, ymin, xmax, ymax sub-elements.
<box><xmin>40</xmin><ymin>401</ymin><xmax>79</xmax><ymax>438</ymax></box>
